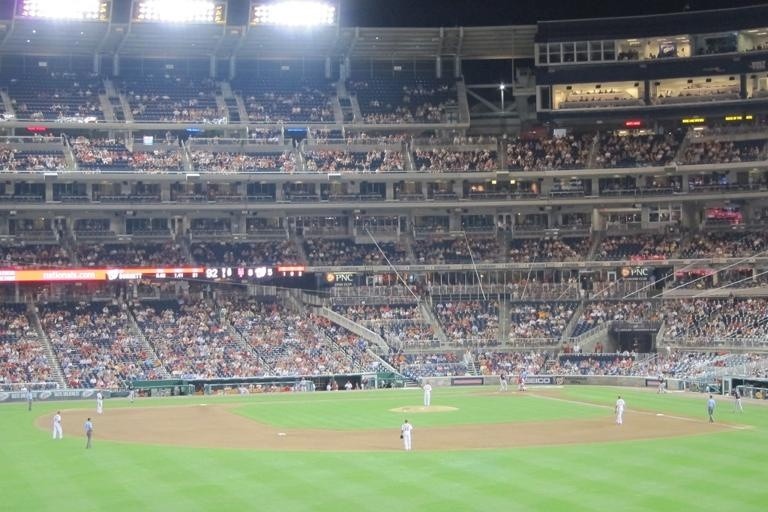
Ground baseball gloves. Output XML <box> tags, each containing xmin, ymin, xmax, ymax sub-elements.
<box><xmin>400</xmin><ymin>434</ymin><xmax>403</xmax><ymax>438</ymax></box>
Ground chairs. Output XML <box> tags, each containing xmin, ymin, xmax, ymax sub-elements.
<box><xmin>0</xmin><ymin>69</ymin><xmax>768</xmax><ymax>398</ymax></box>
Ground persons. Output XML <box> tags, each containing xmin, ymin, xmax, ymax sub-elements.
<box><xmin>84</xmin><ymin>417</ymin><xmax>94</xmax><ymax>450</ymax></box>
<box><xmin>26</xmin><ymin>387</ymin><xmax>35</xmax><ymax>412</ymax></box>
<box><xmin>706</xmin><ymin>394</ymin><xmax>717</xmax><ymax>424</ymax></box>
<box><xmin>0</xmin><ymin>75</ymin><xmax>495</xmax><ymax>174</ymax></box>
<box><xmin>0</xmin><ymin>174</ymin><xmax>767</xmax><ymax>393</ymax></box>
<box><xmin>52</xmin><ymin>410</ymin><xmax>64</xmax><ymax>440</ymax></box>
<box><xmin>733</xmin><ymin>391</ymin><xmax>743</xmax><ymax>414</ymax></box>
<box><xmin>504</xmin><ymin>117</ymin><xmax>768</xmax><ymax>172</ymax></box>
<box><xmin>613</xmin><ymin>395</ymin><xmax>625</xmax><ymax>426</ymax></box>
<box><xmin>423</xmin><ymin>382</ymin><xmax>432</xmax><ymax>407</ymax></box>
<box><xmin>401</xmin><ymin>418</ymin><xmax>413</xmax><ymax>450</ymax></box>
<box><xmin>96</xmin><ymin>390</ymin><xmax>105</xmax><ymax>415</ymax></box>
<box><xmin>129</xmin><ymin>389</ymin><xmax>135</xmax><ymax>409</ymax></box>
